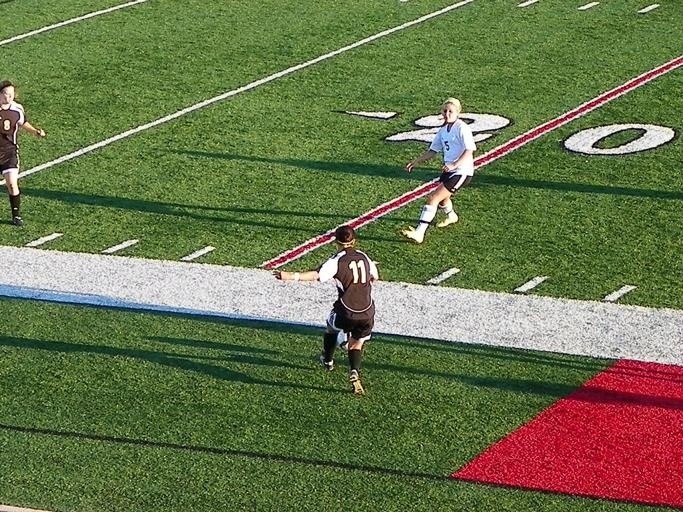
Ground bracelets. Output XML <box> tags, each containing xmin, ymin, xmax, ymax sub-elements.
<box><xmin>293</xmin><ymin>272</ymin><xmax>300</xmax><ymax>281</ymax></box>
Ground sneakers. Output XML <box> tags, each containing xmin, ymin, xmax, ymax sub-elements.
<box><xmin>436</xmin><ymin>213</ymin><xmax>459</xmax><ymax>228</ymax></box>
<box><xmin>349</xmin><ymin>368</ymin><xmax>367</xmax><ymax>396</ymax></box>
<box><xmin>12</xmin><ymin>214</ymin><xmax>24</xmax><ymax>227</ymax></box>
<box><xmin>401</xmin><ymin>230</ymin><xmax>425</xmax><ymax>244</ymax></box>
<box><xmin>319</xmin><ymin>353</ymin><xmax>334</xmax><ymax>371</ymax></box>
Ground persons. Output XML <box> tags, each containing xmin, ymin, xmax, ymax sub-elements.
<box><xmin>398</xmin><ymin>96</ymin><xmax>476</xmax><ymax>243</ymax></box>
<box><xmin>0</xmin><ymin>79</ymin><xmax>46</xmax><ymax>225</ymax></box>
<box><xmin>271</xmin><ymin>225</ymin><xmax>380</xmax><ymax>397</ymax></box>
